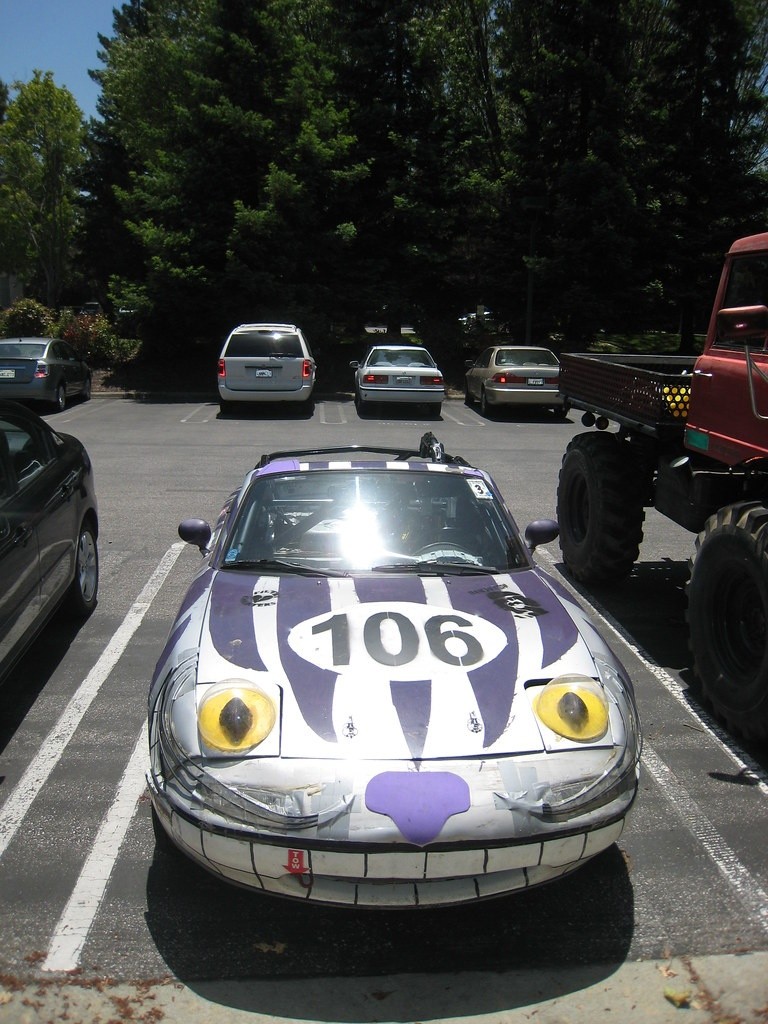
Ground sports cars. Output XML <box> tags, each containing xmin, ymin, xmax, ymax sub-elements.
<box><xmin>142</xmin><ymin>434</ymin><xmax>644</xmax><ymax>912</ymax></box>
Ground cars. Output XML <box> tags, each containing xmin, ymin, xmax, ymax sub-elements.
<box><xmin>462</xmin><ymin>345</ymin><xmax>570</xmax><ymax>416</ymax></box>
<box><xmin>0</xmin><ymin>398</ymin><xmax>102</xmax><ymax>697</ymax></box>
<box><xmin>348</xmin><ymin>346</ymin><xmax>445</xmax><ymax>417</ymax></box>
<box><xmin>0</xmin><ymin>336</ymin><xmax>94</xmax><ymax>412</ymax></box>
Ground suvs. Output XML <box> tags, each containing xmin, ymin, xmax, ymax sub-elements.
<box><xmin>217</xmin><ymin>322</ymin><xmax>318</xmax><ymax>416</ymax></box>
<box><xmin>552</xmin><ymin>229</ymin><xmax>768</xmax><ymax>755</ymax></box>
